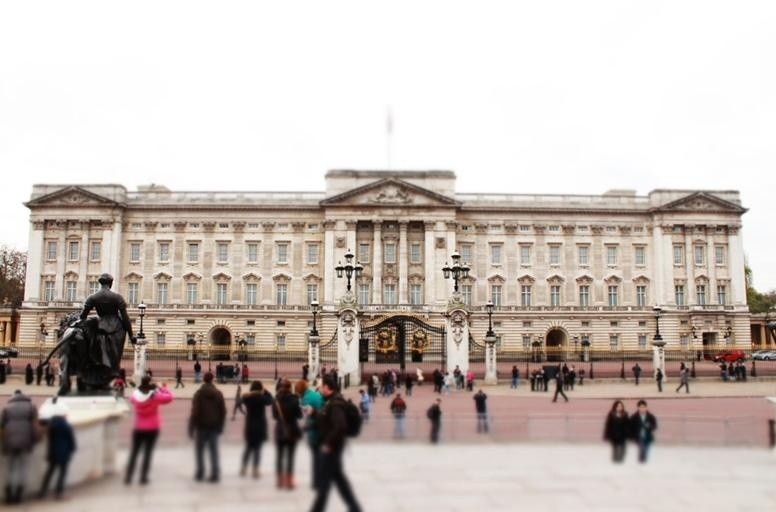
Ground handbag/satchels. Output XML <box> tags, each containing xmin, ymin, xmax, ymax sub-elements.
<box><xmin>330</xmin><ymin>397</ymin><xmax>358</xmax><ymax>435</ymax></box>
<box><xmin>277</xmin><ymin>415</ymin><xmax>300</xmax><ymax>441</ymax></box>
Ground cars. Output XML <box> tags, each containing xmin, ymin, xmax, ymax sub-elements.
<box><xmin>752</xmin><ymin>350</ymin><xmax>775</xmax><ymax>361</ymax></box>
<box><xmin>0</xmin><ymin>344</ymin><xmax>19</xmax><ymax>357</ymax></box>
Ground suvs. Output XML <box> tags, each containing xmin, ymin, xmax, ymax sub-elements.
<box><xmin>711</xmin><ymin>349</ymin><xmax>745</xmax><ymax>363</ymax></box>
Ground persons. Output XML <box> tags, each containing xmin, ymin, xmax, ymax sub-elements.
<box><xmin>175</xmin><ymin>367</ymin><xmax>185</xmax><ymax>388</ymax></box>
<box><xmin>232</xmin><ymin>384</ymin><xmax>245</xmax><ymax>419</ymax></box>
<box><xmin>294</xmin><ymin>379</ymin><xmax>324</xmax><ymax>487</ymax></box>
<box><xmin>390</xmin><ymin>394</ymin><xmax>406</xmax><ymax>437</ymax></box>
<box><xmin>474</xmin><ymin>390</ymin><xmax>489</xmax><ymax>432</ymax></box>
<box><xmin>218</xmin><ymin>362</ymin><xmax>225</xmax><ymax>384</ymax></box>
<box><xmin>553</xmin><ymin>373</ymin><xmax>569</xmax><ymax>403</ymax></box>
<box><xmin>433</xmin><ymin>364</ymin><xmax>474</xmax><ymax>394</ymax></box>
<box><xmin>303</xmin><ymin>365</ymin><xmax>309</xmax><ymax>379</ymax></box>
<box><xmin>429</xmin><ymin>398</ymin><xmax>441</xmax><ymax>442</ymax></box>
<box><xmin>240</xmin><ymin>381</ymin><xmax>273</xmax><ymax>478</ymax></box>
<box><xmin>511</xmin><ymin>365</ymin><xmax>519</xmax><ymax>389</ymax></box>
<box><xmin>126</xmin><ymin>377</ymin><xmax>172</xmax><ymax>485</ymax></box>
<box><xmin>676</xmin><ymin>363</ymin><xmax>690</xmax><ymax>394</ymax></box>
<box><xmin>562</xmin><ymin>363</ymin><xmax>585</xmax><ymax>390</ymax></box>
<box><xmin>26</xmin><ymin>363</ymin><xmax>62</xmax><ymax>387</ymax></box>
<box><xmin>303</xmin><ymin>376</ymin><xmax>361</xmax><ymax>512</ymax></box>
<box><xmin>367</xmin><ymin>369</ymin><xmax>413</xmax><ymax>402</ymax></box>
<box><xmin>359</xmin><ymin>390</ymin><xmax>370</xmax><ymax>423</ymax></box>
<box><xmin>233</xmin><ymin>363</ymin><xmax>249</xmax><ymax>384</ymax></box>
<box><xmin>604</xmin><ymin>400</ymin><xmax>657</xmax><ymax>462</ymax></box>
<box><xmin>530</xmin><ymin>369</ymin><xmax>549</xmax><ymax>391</ymax></box>
<box><xmin>39</xmin><ymin>408</ymin><xmax>76</xmax><ymax>502</ymax></box>
<box><xmin>322</xmin><ymin>364</ymin><xmax>338</xmax><ymax>380</ymax></box>
<box><xmin>656</xmin><ymin>368</ymin><xmax>663</xmax><ymax>393</ymax></box>
<box><xmin>0</xmin><ymin>390</ymin><xmax>40</xmax><ymax>504</ymax></box>
<box><xmin>194</xmin><ymin>361</ymin><xmax>201</xmax><ymax>382</ymax></box>
<box><xmin>188</xmin><ymin>372</ymin><xmax>226</xmax><ymax>483</ymax></box>
<box><xmin>273</xmin><ymin>378</ymin><xmax>303</xmax><ymax>490</ymax></box>
<box><xmin>719</xmin><ymin>360</ymin><xmax>757</xmax><ymax>381</ymax></box>
<box><xmin>80</xmin><ymin>274</ymin><xmax>137</xmax><ymax>371</ymax></box>
<box><xmin>632</xmin><ymin>363</ymin><xmax>642</xmax><ymax>385</ymax></box>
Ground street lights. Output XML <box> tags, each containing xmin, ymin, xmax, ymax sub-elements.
<box><xmin>308</xmin><ymin>298</ymin><xmax>321</xmax><ymax>338</ymax></box>
<box><xmin>441</xmin><ymin>250</ymin><xmax>471</xmax><ymax>292</ymax></box>
<box><xmin>197</xmin><ymin>331</ymin><xmax>204</xmax><ymax>352</ymax></box>
<box><xmin>132</xmin><ymin>301</ymin><xmax>145</xmax><ymax>337</ymax></box>
<box><xmin>573</xmin><ymin>333</ymin><xmax>579</xmax><ymax>355</ymax></box>
<box><xmin>234</xmin><ymin>331</ymin><xmax>239</xmax><ymax>353</ymax></box>
<box><xmin>483</xmin><ymin>300</ymin><xmax>494</xmax><ymax>336</ymax></box>
<box><xmin>651</xmin><ymin>304</ymin><xmax>662</xmax><ymax>340</ymax></box>
<box><xmin>335</xmin><ymin>250</ymin><xmax>362</xmax><ymax>289</ymax></box>
<box><xmin>538</xmin><ymin>333</ymin><xmax>545</xmax><ymax>354</ymax></box>
